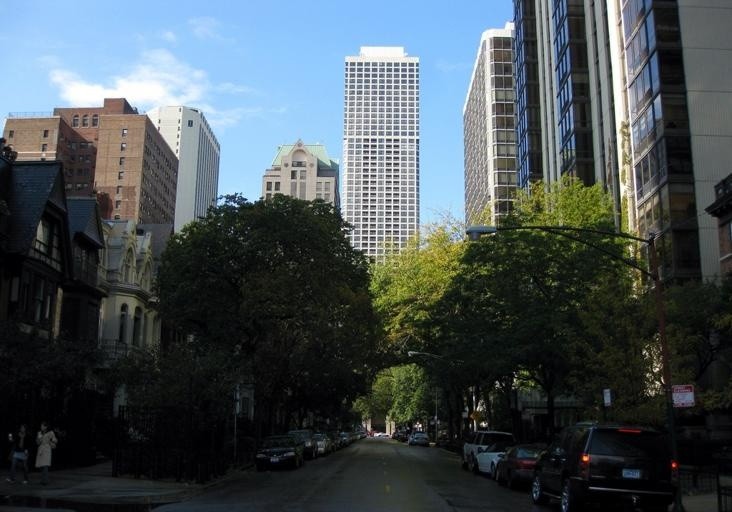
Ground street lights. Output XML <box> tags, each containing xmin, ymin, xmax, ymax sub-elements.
<box><xmin>463</xmin><ymin>226</ymin><xmax>687</xmax><ymax>512</ymax></box>
<box><xmin>405</xmin><ymin>350</ymin><xmax>478</xmax><ymax>432</ymax></box>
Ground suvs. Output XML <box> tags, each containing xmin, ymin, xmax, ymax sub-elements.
<box><xmin>254</xmin><ymin>433</ymin><xmax>306</xmax><ymax>472</ymax></box>
<box><xmin>406</xmin><ymin>430</ymin><xmax>430</xmax><ymax>447</ymax></box>
<box><xmin>528</xmin><ymin>420</ymin><xmax>671</xmax><ymax>511</ymax></box>
<box><xmin>457</xmin><ymin>428</ymin><xmax>515</xmax><ymax>467</ymax></box>
<box><xmin>286</xmin><ymin>428</ymin><xmax>319</xmax><ymax>461</ymax></box>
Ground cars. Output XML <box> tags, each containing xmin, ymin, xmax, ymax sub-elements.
<box><xmin>390</xmin><ymin>428</ymin><xmax>411</xmax><ymax>441</ymax></box>
<box><xmin>491</xmin><ymin>443</ymin><xmax>540</xmax><ymax>489</ymax></box>
<box><xmin>470</xmin><ymin>440</ymin><xmax>520</xmax><ymax>475</ymax></box>
<box><xmin>315</xmin><ymin>429</ymin><xmax>368</xmax><ymax>456</ymax></box>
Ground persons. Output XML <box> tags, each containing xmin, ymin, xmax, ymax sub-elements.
<box><xmin>4</xmin><ymin>423</ymin><xmax>30</xmax><ymax>485</ymax></box>
<box><xmin>33</xmin><ymin>420</ymin><xmax>58</xmax><ymax>487</ymax></box>
